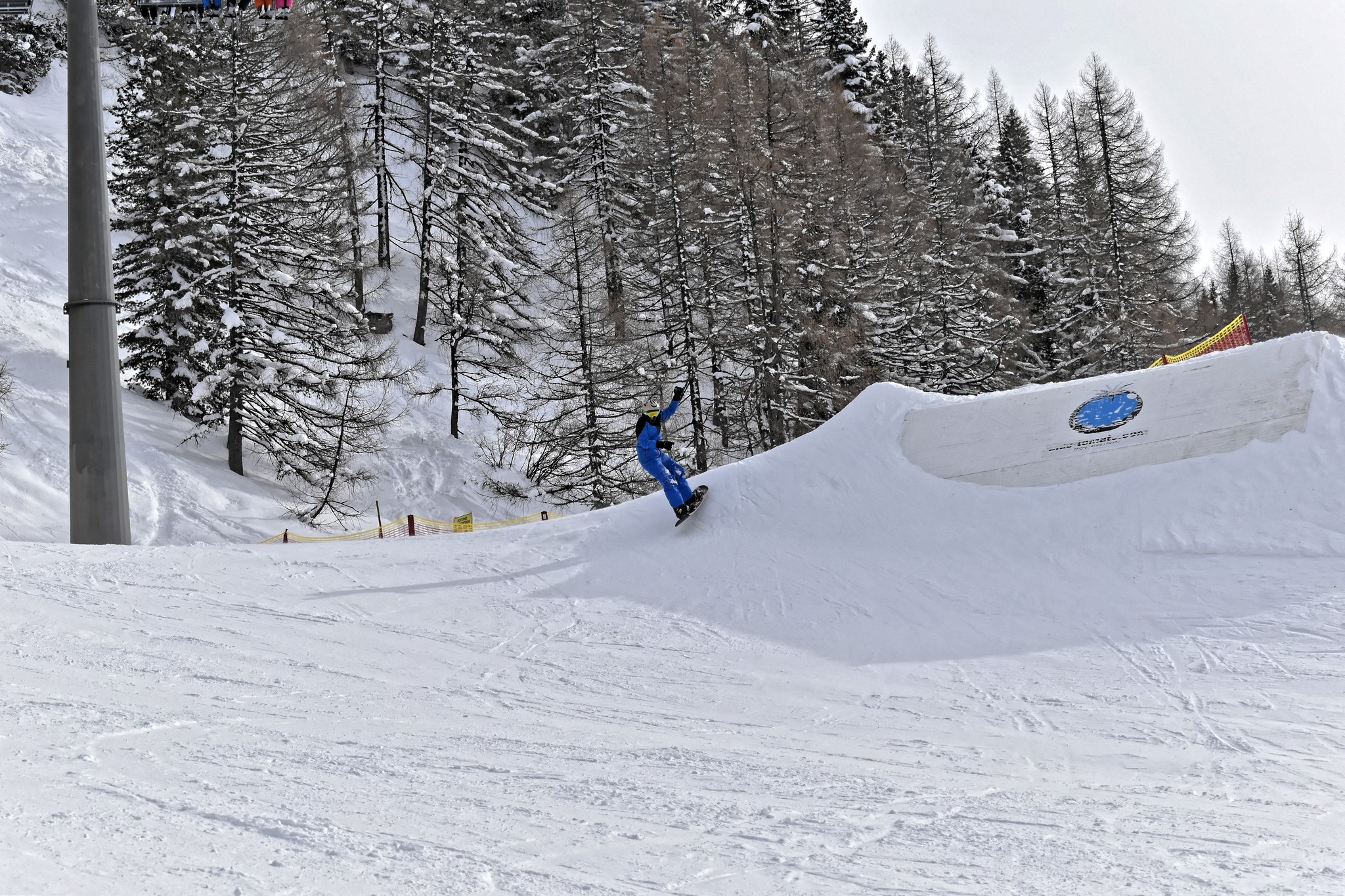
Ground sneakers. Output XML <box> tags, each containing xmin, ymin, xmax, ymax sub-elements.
<box><xmin>674</xmin><ymin>503</ymin><xmax>696</xmax><ymax>518</ymax></box>
<box><xmin>687</xmin><ymin>492</ymin><xmax>704</xmax><ymax>505</ymax></box>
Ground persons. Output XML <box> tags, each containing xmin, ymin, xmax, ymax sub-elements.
<box><xmin>635</xmin><ymin>385</ymin><xmax>700</xmax><ymax>520</ymax></box>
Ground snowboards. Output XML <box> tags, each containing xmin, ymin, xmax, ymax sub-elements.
<box><xmin>676</xmin><ymin>485</ymin><xmax>709</xmax><ymax>528</ymax></box>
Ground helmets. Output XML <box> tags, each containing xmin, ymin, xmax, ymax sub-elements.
<box><xmin>642</xmin><ymin>400</ymin><xmax>660</xmax><ymax>412</ymax></box>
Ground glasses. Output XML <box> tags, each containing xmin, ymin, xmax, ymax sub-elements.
<box><xmin>647</xmin><ymin>411</ymin><xmax>659</xmax><ymax>417</ymax></box>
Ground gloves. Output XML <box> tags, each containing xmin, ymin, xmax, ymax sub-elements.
<box><xmin>672</xmin><ymin>385</ymin><xmax>685</xmax><ymax>401</ymax></box>
<box><xmin>656</xmin><ymin>440</ymin><xmax>675</xmax><ymax>451</ymax></box>
